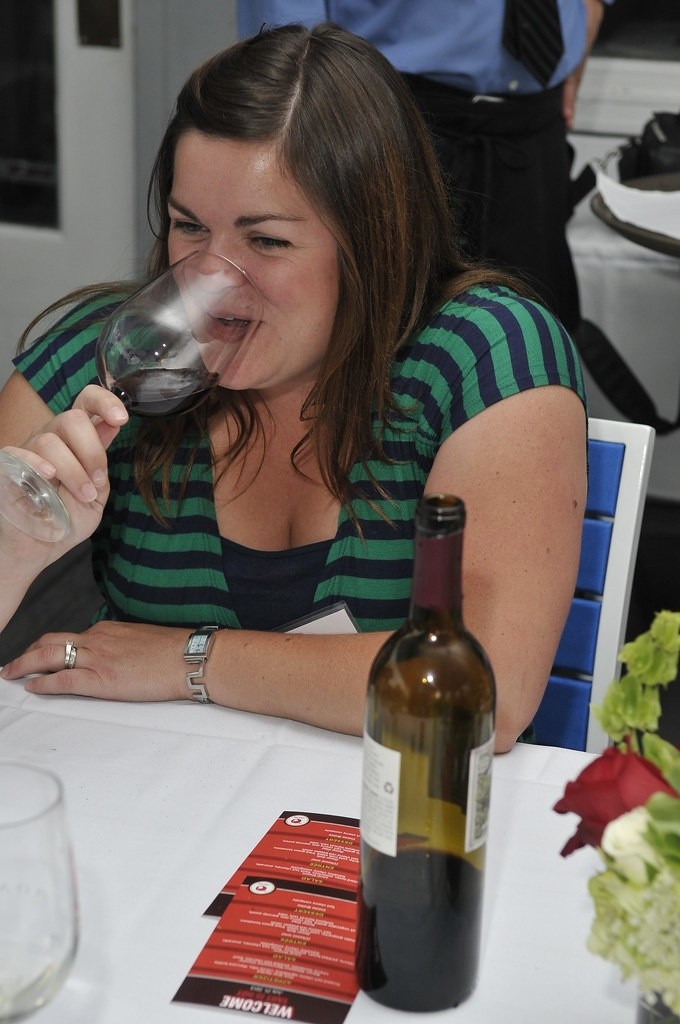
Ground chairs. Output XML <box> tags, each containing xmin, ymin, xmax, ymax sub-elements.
<box><xmin>515</xmin><ymin>413</ymin><xmax>654</xmax><ymax>763</ymax></box>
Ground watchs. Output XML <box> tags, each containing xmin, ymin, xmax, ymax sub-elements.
<box><xmin>184</xmin><ymin>626</ymin><xmax>228</xmax><ymax>705</ymax></box>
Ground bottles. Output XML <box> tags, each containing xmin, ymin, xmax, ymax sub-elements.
<box><xmin>357</xmin><ymin>492</ymin><xmax>497</xmax><ymax>1012</ymax></box>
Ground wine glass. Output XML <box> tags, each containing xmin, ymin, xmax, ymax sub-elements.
<box><xmin>0</xmin><ymin>250</ymin><xmax>262</xmax><ymax>543</ymax></box>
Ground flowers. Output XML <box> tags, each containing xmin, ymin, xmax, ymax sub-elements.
<box><xmin>552</xmin><ymin>607</ymin><xmax>679</xmax><ymax>1016</ymax></box>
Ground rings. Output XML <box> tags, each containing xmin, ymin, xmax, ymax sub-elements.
<box><xmin>69</xmin><ymin>647</ymin><xmax>77</xmax><ymax>669</ymax></box>
<box><xmin>65</xmin><ymin>640</ymin><xmax>74</xmax><ymax>669</ymax></box>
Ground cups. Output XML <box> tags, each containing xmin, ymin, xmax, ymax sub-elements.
<box><xmin>0</xmin><ymin>762</ymin><xmax>79</xmax><ymax>1023</ymax></box>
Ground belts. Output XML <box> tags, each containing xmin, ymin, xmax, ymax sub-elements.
<box><xmin>401</xmin><ymin>74</ymin><xmax>564</xmax><ymax>135</ymax></box>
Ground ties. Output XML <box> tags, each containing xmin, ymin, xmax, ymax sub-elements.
<box><xmin>503</xmin><ymin>2</ymin><xmax>564</xmax><ymax>89</ymax></box>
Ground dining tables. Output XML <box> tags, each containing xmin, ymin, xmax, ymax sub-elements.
<box><xmin>566</xmin><ymin>130</ymin><xmax>680</xmax><ymax>504</ymax></box>
<box><xmin>0</xmin><ymin>661</ymin><xmax>680</xmax><ymax>1021</ymax></box>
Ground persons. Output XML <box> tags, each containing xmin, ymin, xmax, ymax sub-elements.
<box><xmin>237</xmin><ymin>1</ymin><xmax>607</xmax><ymax>349</ymax></box>
<box><xmin>0</xmin><ymin>22</ymin><xmax>589</xmax><ymax>755</ymax></box>
